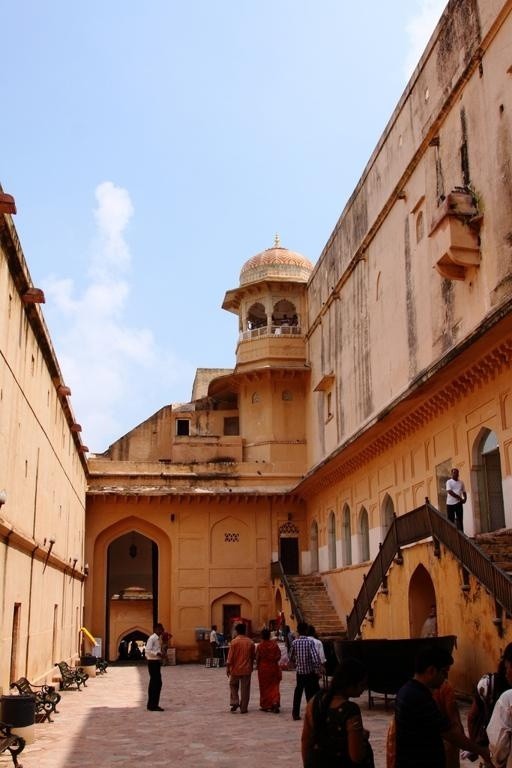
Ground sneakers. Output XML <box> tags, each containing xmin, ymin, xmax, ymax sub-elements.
<box><xmin>147</xmin><ymin>705</ymin><xmax>164</xmax><ymax>712</ymax></box>
<box><xmin>259</xmin><ymin>707</ymin><xmax>280</xmax><ymax>713</ymax></box>
<box><xmin>231</xmin><ymin>703</ymin><xmax>248</xmax><ymax>713</ymax></box>
<box><xmin>292</xmin><ymin>715</ymin><xmax>303</xmax><ymax>721</ymax></box>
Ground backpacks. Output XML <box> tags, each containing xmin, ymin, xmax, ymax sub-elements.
<box><xmin>307</xmin><ymin>687</ymin><xmax>375</xmax><ymax>768</ymax></box>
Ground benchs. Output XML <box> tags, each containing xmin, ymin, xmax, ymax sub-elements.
<box><xmin>0</xmin><ymin>718</ymin><xmax>29</xmax><ymax>766</ymax></box>
<box><xmin>11</xmin><ymin>675</ymin><xmax>63</xmax><ymax>723</ymax></box>
<box><xmin>95</xmin><ymin>657</ymin><xmax>109</xmax><ymax>675</ymax></box>
<box><xmin>53</xmin><ymin>659</ymin><xmax>88</xmax><ymax>691</ymax></box>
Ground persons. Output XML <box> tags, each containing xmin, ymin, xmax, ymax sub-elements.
<box><xmin>159</xmin><ymin>629</ymin><xmax>173</xmax><ymax>666</ymax></box>
<box><xmin>280</xmin><ymin>315</ymin><xmax>291</xmax><ymax>326</ymax></box>
<box><xmin>144</xmin><ymin>624</ymin><xmax>165</xmax><ymax>711</ymax></box>
<box><xmin>128</xmin><ymin>640</ymin><xmax>145</xmax><ymax>660</ymax></box>
<box><xmin>290</xmin><ymin>315</ymin><xmax>298</xmax><ymax>334</ymax></box>
<box><xmin>446</xmin><ymin>469</ymin><xmax>467</xmax><ymax>533</ymax></box>
<box><xmin>272</xmin><ymin>316</ymin><xmax>276</xmax><ymax>325</ymax></box>
